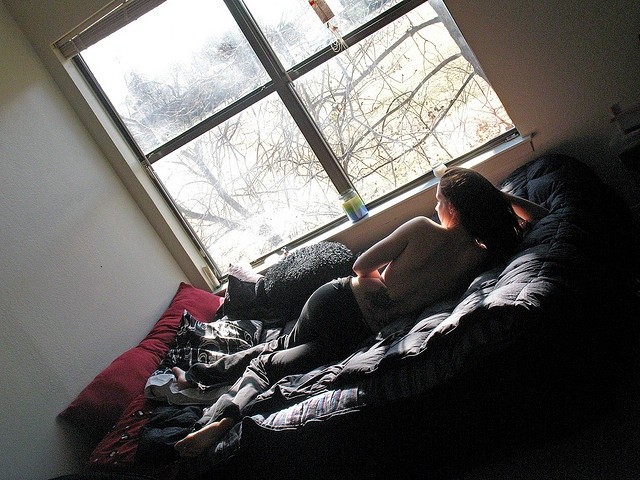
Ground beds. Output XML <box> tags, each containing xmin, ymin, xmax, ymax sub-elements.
<box><xmin>84</xmin><ymin>150</ymin><xmax>630</xmax><ymax>480</ymax></box>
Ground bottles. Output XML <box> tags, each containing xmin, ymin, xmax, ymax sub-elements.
<box><xmin>430</xmin><ymin>160</ymin><xmax>449</xmax><ymax>179</ymax></box>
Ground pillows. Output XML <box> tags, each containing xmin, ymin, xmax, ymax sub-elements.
<box><xmin>58</xmin><ymin>282</ymin><xmax>225</xmax><ymax>439</ymax></box>
<box><xmin>210</xmin><ymin>263</ymin><xmax>285</xmax><ymax>331</ymax></box>
<box><xmin>148</xmin><ymin>310</ymin><xmax>262</xmax><ymax>404</ymax></box>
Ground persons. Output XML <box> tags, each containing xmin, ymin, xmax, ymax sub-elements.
<box><xmin>170</xmin><ymin>165</ymin><xmax>552</xmax><ymax>460</ymax></box>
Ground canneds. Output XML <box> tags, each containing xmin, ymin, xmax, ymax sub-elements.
<box><xmin>432</xmin><ymin>161</ymin><xmax>447</xmax><ymax>176</ymax></box>
